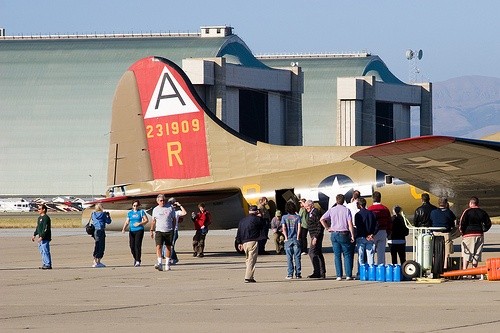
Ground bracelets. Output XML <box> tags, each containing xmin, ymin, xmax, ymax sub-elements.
<box><xmin>150</xmin><ymin>230</ymin><xmax>153</xmax><ymax>231</ymax></box>
<box><xmin>179</xmin><ymin>205</ymin><xmax>182</xmax><ymax>207</ymax></box>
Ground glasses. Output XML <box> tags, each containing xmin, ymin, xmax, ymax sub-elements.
<box><xmin>38</xmin><ymin>208</ymin><xmax>42</xmax><ymax>210</ymax></box>
<box><xmin>133</xmin><ymin>205</ymin><xmax>139</xmax><ymax>208</ymax></box>
<box><xmin>158</xmin><ymin>199</ymin><xmax>165</xmax><ymax>202</ymax></box>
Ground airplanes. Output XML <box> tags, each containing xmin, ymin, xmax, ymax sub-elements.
<box><xmin>82</xmin><ymin>56</ymin><xmax>500</xmax><ymax>231</ymax></box>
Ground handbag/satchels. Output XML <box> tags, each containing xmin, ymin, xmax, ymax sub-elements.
<box><xmin>85</xmin><ymin>222</ymin><xmax>95</xmax><ymax>235</ymax></box>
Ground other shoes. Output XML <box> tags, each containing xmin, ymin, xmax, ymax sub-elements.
<box><xmin>38</xmin><ymin>265</ymin><xmax>53</xmax><ymax>270</ymax></box>
<box><xmin>155</xmin><ymin>263</ymin><xmax>163</xmax><ymax>271</ymax></box>
<box><xmin>346</xmin><ymin>277</ymin><xmax>353</xmax><ymax>280</ymax></box>
<box><xmin>245</xmin><ymin>278</ymin><xmax>256</xmax><ymax>283</ymax></box>
<box><xmin>165</xmin><ymin>265</ymin><xmax>172</xmax><ymax>271</ymax></box>
<box><xmin>296</xmin><ymin>276</ymin><xmax>302</xmax><ymax>279</ymax></box>
<box><xmin>170</xmin><ymin>259</ymin><xmax>179</xmax><ymax>264</ymax></box>
<box><xmin>307</xmin><ymin>274</ymin><xmax>326</xmax><ymax>279</ymax></box>
<box><xmin>197</xmin><ymin>253</ymin><xmax>204</xmax><ymax>257</ymax></box>
<box><xmin>285</xmin><ymin>276</ymin><xmax>293</xmax><ymax>279</ymax></box>
<box><xmin>336</xmin><ymin>277</ymin><xmax>342</xmax><ymax>280</ymax></box>
<box><xmin>93</xmin><ymin>262</ymin><xmax>105</xmax><ymax>267</ymax></box>
<box><xmin>135</xmin><ymin>261</ymin><xmax>140</xmax><ymax>267</ymax></box>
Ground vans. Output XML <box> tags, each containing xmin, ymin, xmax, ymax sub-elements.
<box><xmin>12</xmin><ymin>203</ymin><xmax>29</xmax><ymax>212</ymax></box>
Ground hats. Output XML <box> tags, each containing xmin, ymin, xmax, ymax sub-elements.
<box><xmin>298</xmin><ymin>198</ymin><xmax>306</xmax><ymax>202</ymax></box>
<box><xmin>168</xmin><ymin>198</ymin><xmax>176</xmax><ymax>204</ymax></box>
<box><xmin>276</xmin><ymin>211</ymin><xmax>282</xmax><ymax>216</ymax></box>
<box><xmin>249</xmin><ymin>205</ymin><xmax>259</xmax><ymax>211</ymax></box>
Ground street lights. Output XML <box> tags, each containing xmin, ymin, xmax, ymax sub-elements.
<box><xmin>88</xmin><ymin>174</ymin><xmax>93</xmax><ymax>201</ymax></box>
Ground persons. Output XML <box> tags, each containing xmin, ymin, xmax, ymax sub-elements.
<box><xmin>281</xmin><ymin>202</ymin><xmax>301</xmax><ymax>279</ymax></box>
<box><xmin>32</xmin><ymin>204</ymin><xmax>52</xmax><ymax>269</ymax></box>
<box><xmin>355</xmin><ymin>198</ymin><xmax>378</xmax><ymax>280</ymax></box>
<box><xmin>258</xmin><ymin>191</ymin><xmax>457</xmax><ymax>269</ymax></box>
<box><xmin>320</xmin><ymin>194</ymin><xmax>355</xmax><ymax>281</ymax></box>
<box><xmin>150</xmin><ymin>194</ymin><xmax>176</xmax><ymax>271</ymax></box>
<box><xmin>237</xmin><ymin>206</ymin><xmax>265</xmax><ymax>282</ymax></box>
<box><xmin>192</xmin><ymin>202</ymin><xmax>211</xmax><ymax>257</ymax></box>
<box><xmin>122</xmin><ymin>200</ymin><xmax>148</xmax><ymax>266</ymax></box>
<box><xmin>459</xmin><ymin>197</ymin><xmax>492</xmax><ymax>278</ymax></box>
<box><xmin>92</xmin><ymin>204</ymin><xmax>111</xmax><ymax>267</ymax></box>
<box><xmin>162</xmin><ymin>197</ymin><xmax>187</xmax><ymax>264</ymax></box>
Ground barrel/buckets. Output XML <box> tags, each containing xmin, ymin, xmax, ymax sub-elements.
<box><xmin>376</xmin><ymin>263</ymin><xmax>385</xmax><ymax>282</ymax></box>
<box><xmin>385</xmin><ymin>264</ymin><xmax>393</xmax><ymax>282</ymax></box>
<box><xmin>393</xmin><ymin>265</ymin><xmax>401</xmax><ymax>282</ymax></box>
<box><xmin>368</xmin><ymin>264</ymin><xmax>376</xmax><ymax>281</ymax></box>
<box><xmin>360</xmin><ymin>262</ymin><xmax>367</xmax><ymax>281</ymax></box>
